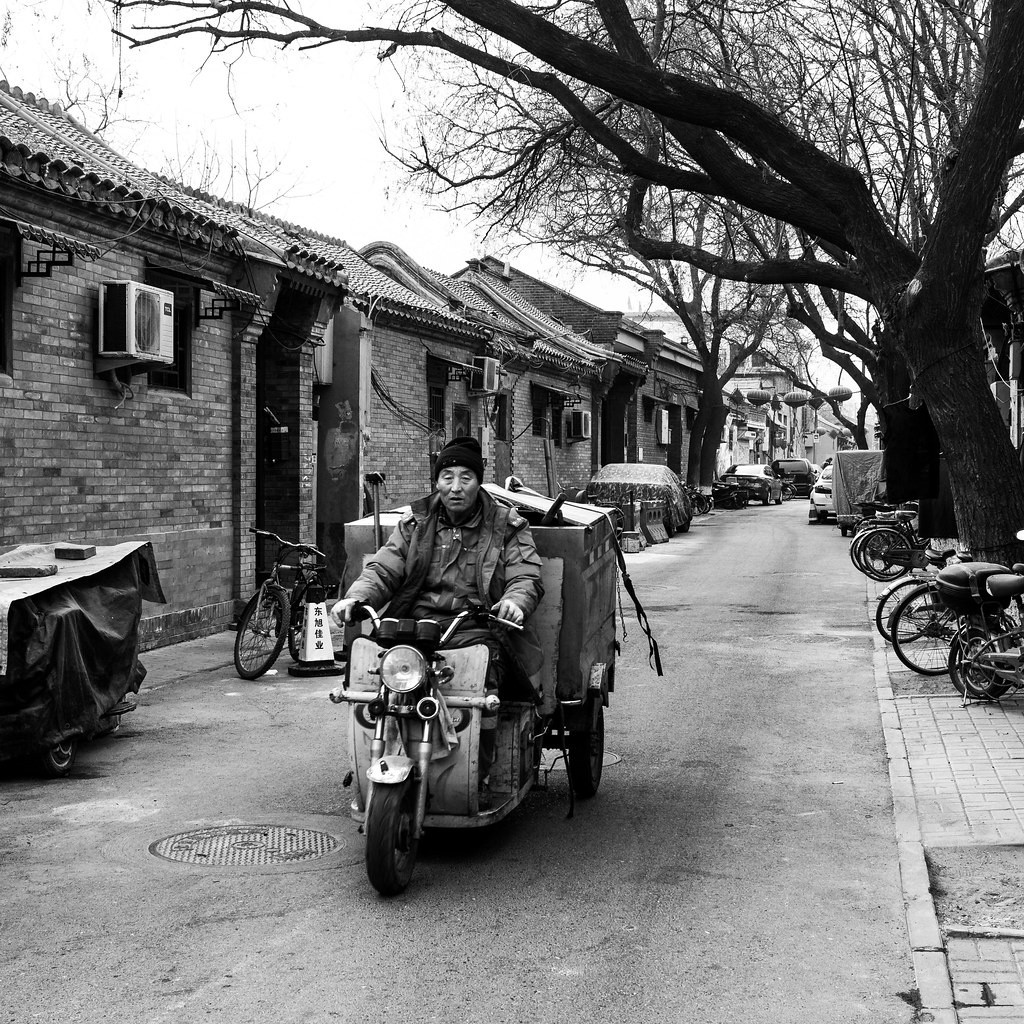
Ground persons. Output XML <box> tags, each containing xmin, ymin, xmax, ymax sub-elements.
<box><xmin>330</xmin><ymin>436</ymin><xmax>545</xmax><ymax>811</ymax></box>
<box><xmin>821</xmin><ymin>457</ymin><xmax>833</xmax><ymax>470</ymax></box>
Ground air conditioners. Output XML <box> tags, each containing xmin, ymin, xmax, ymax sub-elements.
<box><xmin>97</xmin><ymin>278</ymin><xmax>174</xmax><ymax>364</ymax></box>
<box><xmin>571</xmin><ymin>410</ymin><xmax>591</xmax><ymax>439</ymax></box>
<box><xmin>470</xmin><ymin>356</ymin><xmax>501</xmax><ymax>392</ymax></box>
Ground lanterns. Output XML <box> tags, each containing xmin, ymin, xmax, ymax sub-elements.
<box><xmin>747</xmin><ymin>390</ymin><xmax>771</xmax><ymax>407</ymax></box>
<box><xmin>829</xmin><ymin>386</ymin><xmax>852</xmax><ymax>402</ymax></box>
<box><xmin>783</xmin><ymin>392</ymin><xmax>807</xmax><ymax>409</ymax></box>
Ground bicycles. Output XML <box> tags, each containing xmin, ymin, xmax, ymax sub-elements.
<box><xmin>681</xmin><ymin>480</ymin><xmax>714</xmax><ymax>517</ymax></box>
<box><xmin>554</xmin><ymin>480</ymin><xmax>627</xmax><ymax>541</ymax></box>
<box><xmin>776</xmin><ymin>474</ymin><xmax>799</xmax><ymax>503</ymax></box>
<box><xmin>848</xmin><ymin>497</ymin><xmax>959</xmax><ymax>583</ymax></box>
<box><xmin>874</xmin><ymin>541</ymin><xmax>1024</xmax><ymax>706</ymax></box>
<box><xmin>710</xmin><ymin>481</ymin><xmax>748</xmax><ymax>512</ymax></box>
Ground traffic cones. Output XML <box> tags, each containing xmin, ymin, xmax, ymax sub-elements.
<box><xmin>287</xmin><ymin>573</ymin><xmax>347</xmax><ymax>678</ymax></box>
<box><xmin>808</xmin><ymin>497</ymin><xmax>821</xmax><ymax>525</ymax></box>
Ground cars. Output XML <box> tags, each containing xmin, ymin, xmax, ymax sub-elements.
<box><xmin>588</xmin><ymin>463</ymin><xmax>692</xmax><ymax>538</ymax></box>
<box><xmin>809</xmin><ymin>464</ymin><xmax>842</xmax><ymax>525</ymax></box>
<box><xmin>720</xmin><ymin>463</ymin><xmax>785</xmax><ymax>505</ymax></box>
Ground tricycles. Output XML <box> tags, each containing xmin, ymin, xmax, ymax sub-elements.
<box><xmin>833</xmin><ymin>449</ymin><xmax>892</xmax><ymax>537</ymax></box>
<box><xmin>0</xmin><ymin>536</ymin><xmax>167</xmax><ymax>775</ymax></box>
<box><xmin>232</xmin><ymin>525</ymin><xmax>333</xmax><ymax>681</ymax></box>
<box><xmin>335</xmin><ymin>464</ymin><xmax>627</xmax><ymax>901</ymax></box>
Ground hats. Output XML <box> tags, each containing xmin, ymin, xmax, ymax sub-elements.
<box><xmin>434</xmin><ymin>435</ymin><xmax>484</xmax><ymax>486</ymax></box>
